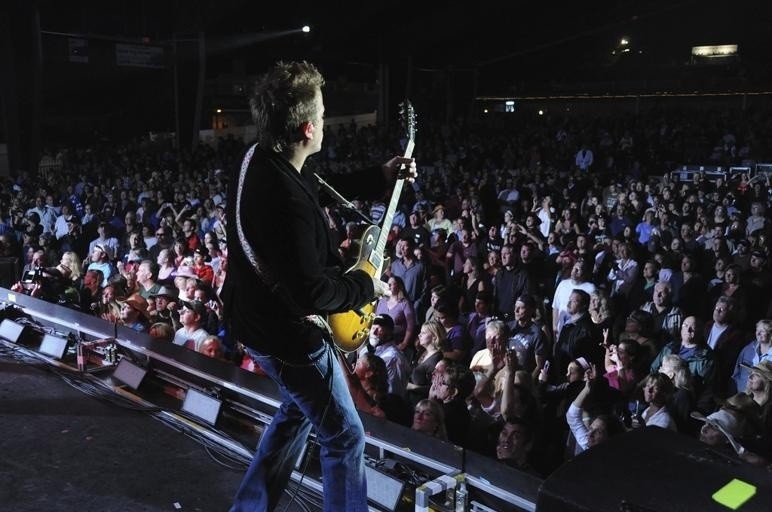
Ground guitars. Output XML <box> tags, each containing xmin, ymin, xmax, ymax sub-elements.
<box><xmin>318</xmin><ymin>99</ymin><xmax>417</xmax><ymax>352</ymax></box>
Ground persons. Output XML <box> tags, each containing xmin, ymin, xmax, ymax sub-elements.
<box><xmin>0</xmin><ymin>118</ymin><xmax>772</xmax><ymax>478</ymax></box>
<box><xmin>222</xmin><ymin>63</ymin><xmax>420</xmax><ymax>511</ymax></box>
